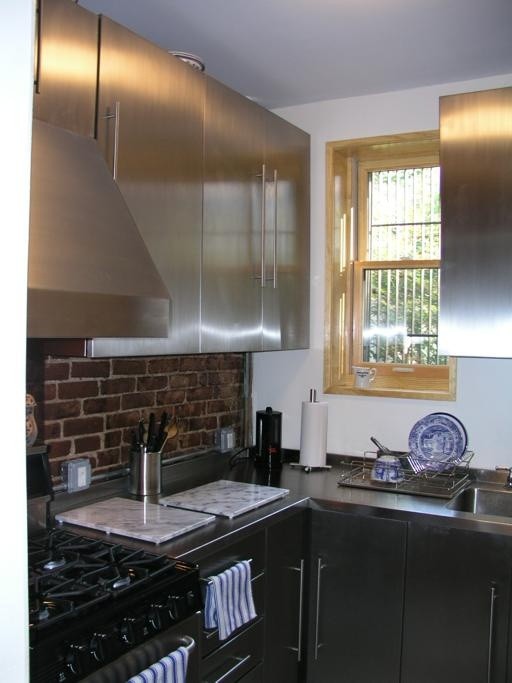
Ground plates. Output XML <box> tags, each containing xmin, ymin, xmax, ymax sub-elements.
<box><xmin>408</xmin><ymin>412</ymin><xmax>468</xmax><ymax>471</ymax></box>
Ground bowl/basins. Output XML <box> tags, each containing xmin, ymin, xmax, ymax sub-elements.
<box><xmin>371</xmin><ymin>455</ymin><xmax>405</xmax><ymax>484</ymax></box>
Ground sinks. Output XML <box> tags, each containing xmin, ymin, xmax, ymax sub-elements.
<box><xmin>444</xmin><ymin>486</ymin><xmax>512</xmax><ymax>517</ymax></box>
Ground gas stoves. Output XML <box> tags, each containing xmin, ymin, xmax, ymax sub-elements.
<box><xmin>29</xmin><ymin>525</ymin><xmax>200</xmax><ymax>682</ymax></box>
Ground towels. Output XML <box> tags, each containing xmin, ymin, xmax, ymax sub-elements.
<box><xmin>127</xmin><ymin>645</ymin><xmax>190</xmax><ymax>682</ymax></box>
<box><xmin>203</xmin><ymin>560</ymin><xmax>259</xmax><ymax>640</ymax></box>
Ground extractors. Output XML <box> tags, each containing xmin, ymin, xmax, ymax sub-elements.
<box><xmin>28</xmin><ymin>118</ymin><xmax>173</xmax><ymax>338</ymax></box>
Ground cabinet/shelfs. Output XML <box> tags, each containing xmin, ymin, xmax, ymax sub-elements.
<box><xmin>310</xmin><ymin>507</ymin><xmax>409</xmax><ymax>683</ymax></box>
<box><xmin>437</xmin><ymin>86</ymin><xmax>512</xmax><ymax>358</ymax></box>
<box><xmin>32</xmin><ymin>0</ymin><xmax>201</xmax><ymax>359</ymax></box>
<box><xmin>32</xmin><ymin>0</ymin><xmax>101</xmax><ymax>141</ymax></box>
<box><xmin>265</xmin><ymin>510</ymin><xmax>310</xmax><ymax>682</ymax></box>
<box><xmin>400</xmin><ymin>514</ymin><xmax>512</xmax><ymax>683</ymax></box>
<box><xmin>200</xmin><ymin>68</ymin><xmax>313</xmax><ymax>355</ymax></box>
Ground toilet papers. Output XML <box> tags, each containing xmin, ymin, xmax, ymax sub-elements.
<box><xmin>299</xmin><ymin>400</ymin><xmax>328</xmax><ymax>467</ymax></box>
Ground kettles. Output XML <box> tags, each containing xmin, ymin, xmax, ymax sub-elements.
<box><xmin>253</xmin><ymin>406</ymin><xmax>283</xmax><ymax>467</ymax></box>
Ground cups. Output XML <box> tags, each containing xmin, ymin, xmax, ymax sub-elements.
<box><xmin>350</xmin><ymin>365</ymin><xmax>377</xmax><ymax>388</ymax></box>
<box><xmin>128</xmin><ymin>450</ymin><xmax>161</xmax><ymax>496</ymax></box>
